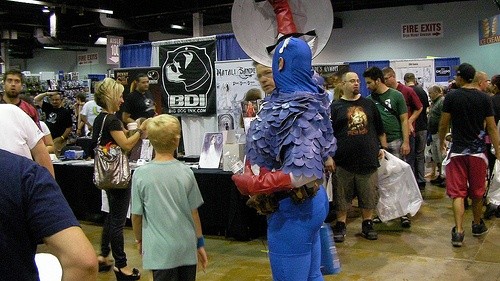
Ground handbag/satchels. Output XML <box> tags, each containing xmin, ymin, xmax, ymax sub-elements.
<box><xmin>377</xmin><ymin>149</ymin><xmax>423</xmax><ymax>222</ymax></box>
<box><xmin>93</xmin><ymin>114</ymin><xmax>131</xmax><ymax>189</ymax></box>
<box><xmin>486</xmin><ymin>160</ymin><xmax>500</xmax><ymax>209</ymax></box>
<box><xmin>319</xmin><ymin>221</ymin><xmax>340</xmax><ymax>275</ymax></box>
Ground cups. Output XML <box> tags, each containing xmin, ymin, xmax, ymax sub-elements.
<box><xmin>127</xmin><ymin>122</ymin><xmax>139</xmax><ymax>137</ymax></box>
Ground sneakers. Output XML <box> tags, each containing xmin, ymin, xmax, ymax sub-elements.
<box><xmin>451</xmin><ymin>226</ymin><xmax>464</xmax><ymax>246</ymax></box>
<box><xmin>472</xmin><ymin>218</ymin><xmax>488</xmax><ymax>235</ymax></box>
<box><xmin>333</xmin><ymin>220</ymin><xmax>347</xmax><ymax>242</ymax></box>
<box><xmin>362</xmin><ymin>219</ymin><xmax>378</xmax><ymax>240</ymax></box>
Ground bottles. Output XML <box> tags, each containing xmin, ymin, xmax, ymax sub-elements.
<box><xmin>224</xmin><ymin>152</ymin><xmax>245</xmax><ymax>176</ymax></box>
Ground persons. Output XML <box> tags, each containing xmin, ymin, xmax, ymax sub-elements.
<box><xmin>438</xmin><ymin>62</ymin><xmax>500</xmax><ymax>246</ymax></box>
<box><xmin>131</xmin><ymin>113</ymin><xmax>208</xmax><ymax>281</ymax></box>
<box><xmin>424</xmin><ymin>85</ymin><xmax>449</xmax><ymax>183</ymax></box>
<box><xmin>0</xmin><ymin>69</ymin><xmax>43</xmax><ymax>133</ymax></box>
<box><xmin>91</xmin><ymin>78</ymin><xmax>149</xmax><ymax>281</ymax></box>
<box><xmin>404</xmin><ymin>73</ymin><xmax>429</xmax><ymax>192</ymax></box>
<box><xmin>122</xmin><ymin>73</ymin><xmax>158</xmax><ymax>137</ymax></box>
<box><xmin>0</xmin><ymin>104</ymin><xmax>55</xmax><ymax>178</ymax></box>
<box><xmin>324</xmin><ymin>71</ymin><xmax>388</xmax><ymax>243</ymax></box>
<box><xmin>381</xmin><ymin>66</ymin><xmax>423</xmax><ymax>174</ymax></box>
<box><xmin>0</xmin><ymin>149</ymin><xmax>99</xmax><ymax>281</ymax></box>
<box><xmin>73</xmin><ymin>92</ymin><xmax>87</xmax><ymax>137</ymax></box>
<box><xmin>79</xmin><ymin>100</ymin><xmax>108</xmax><ymax>136</ymax></box>
<box><xmin>232</xmin><ymin>36</ymin><xmax>337</xmax><ymax>281</ymax></box>
<box><xmin>362</xmin><ymin>66</ymin><xmax>411</xmax><ymax>228</ymax></box>
<box><xmin>33</xmin><ymin>92</ymin><xmax>73</xmax><ymax>158</ymax></box>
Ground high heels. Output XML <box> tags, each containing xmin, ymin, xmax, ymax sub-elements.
<box><xmin>98</xmin><ymin>260</ymin><xmax>114</xmax><ymax>271</ymax></box>
<box><xmin>113</xmin><ymin>266</ymin><xmax>141</xmax><ymax>281</ymax></box>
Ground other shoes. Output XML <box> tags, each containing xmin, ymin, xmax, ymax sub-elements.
<box><xmin>372</xmin><ymin>215</ymin><xmax>382</xmax><ymax>224</ymax></box>
<box><xmin>400</xmin><ymin>215</ymin><xmax>410</xmax><ymax>228</ymax></box>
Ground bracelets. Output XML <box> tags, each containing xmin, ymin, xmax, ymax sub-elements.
<box><xmin>61</xmin><ymin>136</ymin><xmax>64</xmax><ymax>140</ymax></box>
<box><xmin>135</xmin><ymin>238</ymin><xmax>142</xmax><ymax>244</ymax></box>
<box><xmin>197</xmin><ymin>235</ymin><xmax>205</xmax><ymax>249</ymax></box>
<box><xmin>137</xmin><ymin>129</ymin><xmax>143</xmax><ymax>136</ymax></box>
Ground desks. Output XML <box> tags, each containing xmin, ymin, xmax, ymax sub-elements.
<box><xmin>54</xmin><ymin>159</ymin><xmax>268</xmax><ymax>242</ymax></box>
